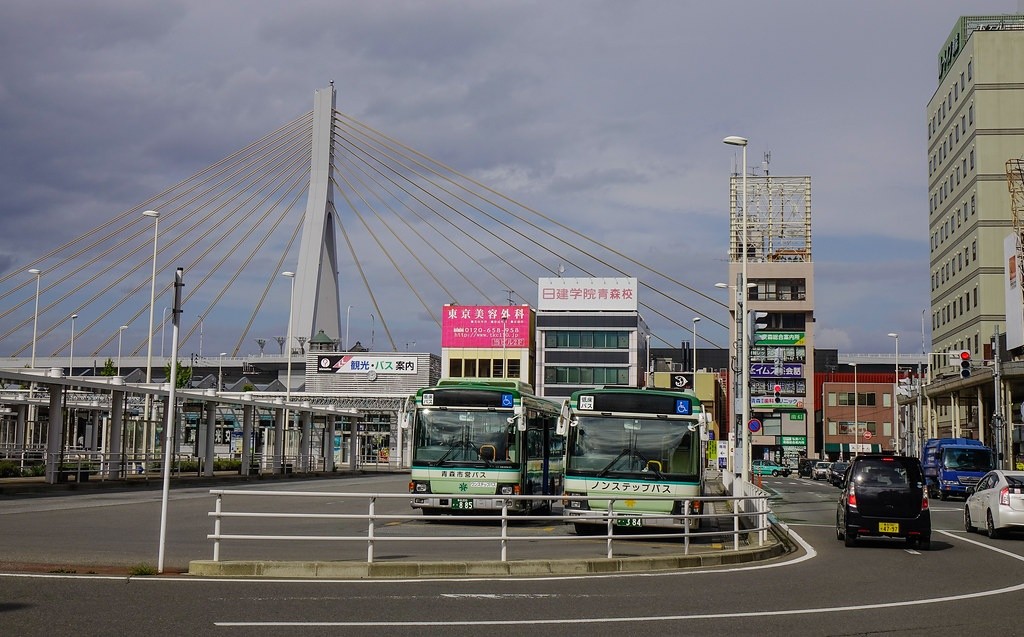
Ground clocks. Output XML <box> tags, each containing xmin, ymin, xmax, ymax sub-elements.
<box><xmin>367</xmin><ymin>370</ymin><xmax>377</xmax><ymax>381</ymax></box>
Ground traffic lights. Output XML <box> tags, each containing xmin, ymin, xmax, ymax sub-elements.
<box><xmin>960</xmin><ymin>349</ymin><xmax>972</xmax><ymax>378</ymax></box>
<box><xmin>749</xmin><ymin>309</ymin><xmax>767</xmax><ymax>348</ymax></box>
<box><xmin>773</xmin><ymin>385</ymin><xmax>782</xmax><ymax>404</ymax></box>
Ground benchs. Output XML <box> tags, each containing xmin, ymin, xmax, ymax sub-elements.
<box><xmin>238</xmin><ymin>464</ymin><xmax>260</xmax><ymax>475</ymax></box>
<box><xmin>57</xmin><ymin>467</ymin><xmax>90</xmax><ymax>483</ymax></box>
<box><xmin>281</xmin><ymin>464</ymin><xmax>292</xmax><ymax>472</ymax></box>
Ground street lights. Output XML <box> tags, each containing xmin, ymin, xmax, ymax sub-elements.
<box><xmin>218</xmin><ymin>352</ymin><xmax>230</xmax><ymax>394</ymax></box>
<box><xmin>68</xmin><ymin>313</ymin><xmax>78</xmax><ymax>389</ymax></box>
<box><xmin>369</xmin><ymin>313</ymin><xmax>376</xmax><ymax>352</ymax></box>
<box><xmin>196</xmin><ymin>314</ymin><xmax>205</xmax><ymax>360</ymax></box>
<box><xmin>887</xmin><ymin>333</ymin><xmax>899</xmax><ymax>454</ymax></box>
<box><xmin>721</xmin><ymin>132</ymin><xmax>752</xmax><ymax>486</ymax></box>
<box><xmin>689</xmin><ymin>313</ymin><xmax>701</xmax><ymax>394</ymax></box>
<box><xmin>163</xmin><ymin>305</ymin><xmax>173</xmax><ymax>359</ymax></box>
<box><xmin>118</xmin><ymin>325</ymin><xmax>130</xmax><ymax>375</ymax></box>
<box><xmin>281</xmin><ymin>271</ymin><xmax>296</xmax><ymax>427</ymax></box>
<box><xmin>848</xmin><ymin>363</ymin><xmax>858</xmax><ymax>458</ymax></box>
<box><xmin>138</xmin><ymin>209</ymin><xmax>162</xmax><ymax>474</ymax></box>
<box><xmin>26</xmin><ymin>268</ymin><xmax>41</xmax><ymax>449</ymax></box>
<box><xmin>715</xmin><ymin>283</ymin><xmax>738</xmax><ymax>384</ymax></box>
<box><xmin>345</xmin><ymin>305</ymin><xmax>353</xmax><ymax>352</ymax></box>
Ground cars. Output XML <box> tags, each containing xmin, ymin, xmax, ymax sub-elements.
<box><xmin>962</xmin><ymin>470</ymin><xmax>1024</xmax><ymax>538</ymax></box>
<box><xmin>811</xmin><ymin>461</ymin><xmax>831</xmax><ymax>480</ymax></box>
<box><xmin>825</xmin><ymin>461</ymin><xmax>850</xmax><ymax>488</ymax></box>
<box><xmin>751</xmin><ymin>459</ymin><xmax>793</xmax><ymax>478</ymax></box>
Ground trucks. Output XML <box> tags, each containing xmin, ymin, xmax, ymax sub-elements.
<box><xmin>921</xmin><ymin>438</ymin><xmax>1004</xmax><ymax>501</ymax></box>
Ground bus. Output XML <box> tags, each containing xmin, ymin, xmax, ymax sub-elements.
<box><xmin>398</xmin><ymin>377</ymin><xmax>565</xmax><ymax>521</ymax></box>
<box><xmin>555</xmin><ymin>384</ymin><xmax>711</xmax><ymax>542</ymax></box>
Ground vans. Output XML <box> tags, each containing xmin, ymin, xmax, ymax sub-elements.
<box><xmin>835</xmin><ymin>452</ymin><xmax>934</xmax><ymax>551</ymax></box>
<box><xmin>797</xmin><ymin>458</ymin><xmax>822</xmax><ymax>478</ymax></box>
